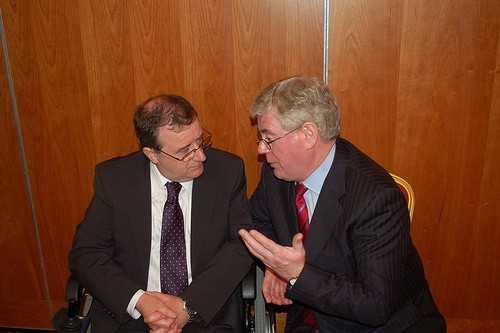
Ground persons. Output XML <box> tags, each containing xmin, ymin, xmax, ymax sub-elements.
<box><xmin>239</xmin><ymin>76</ymin><xmax>446</xmax><ymax>333</ymax></box>
<box><xmin>69</xmin><ymin>94</ymin><xmax>254</xmax><ymax>333</ymax></box>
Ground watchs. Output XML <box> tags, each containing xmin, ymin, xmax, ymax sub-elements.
<box><xmin>289</xmin><ymin>278</ymin><xmax>299</xmax><ymax>289</ymax></box>
<box><xmin>183</xmin><ymin>300</ymin><xmax>197</xmax><ymax>324</ymax></box>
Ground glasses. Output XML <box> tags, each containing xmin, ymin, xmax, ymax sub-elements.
<box><xmin>150</xmin><ymin>128</ymin><xmax>212</xmax><ymax>162</ymax></box>
<box><xmin>256</xmin><ymin>125</ymin><xmax>301</xmax><ymax>150</ymax></box>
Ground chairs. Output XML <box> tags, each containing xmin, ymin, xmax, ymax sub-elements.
<box><xmin>61</xmin><ymin>173</ymin><xmax>416</xmax><ymax>333</ymax></box>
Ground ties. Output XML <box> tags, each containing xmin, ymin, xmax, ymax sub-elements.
<box><xmin>294</xmin><ymin>182</ymin><xmax>315</xmax><ymax>324</ymax></box>
<box><xmin>160</xmin><ymin>182</ymin><xmax>187</xmax><ymax>296</ymax></box>
